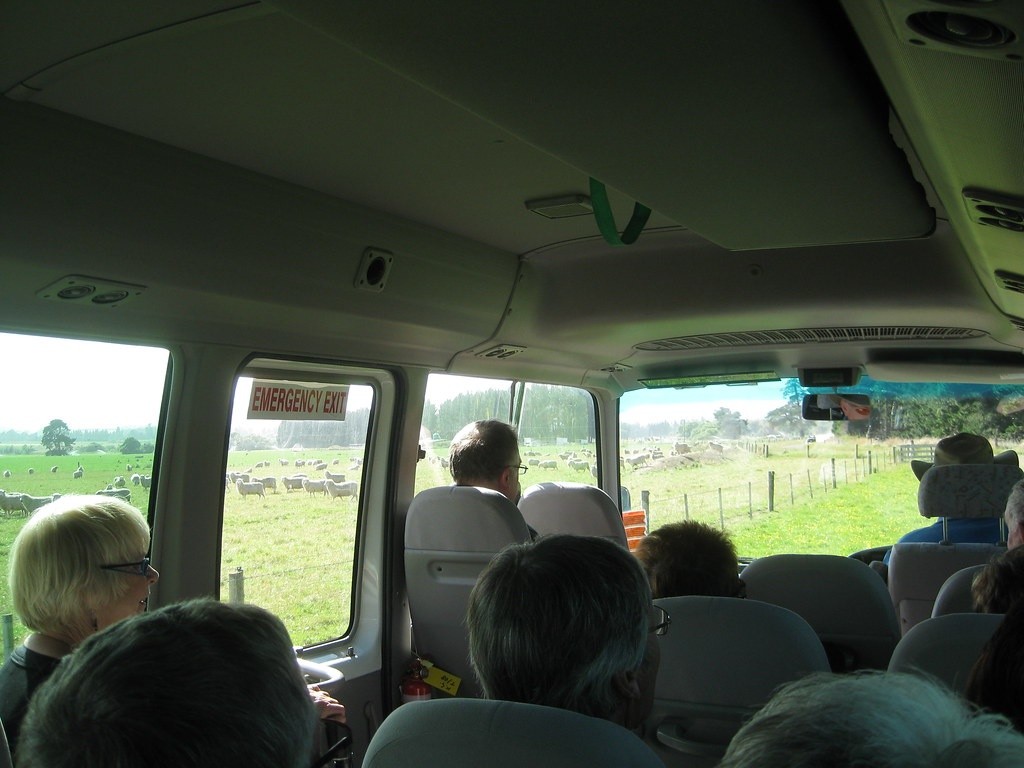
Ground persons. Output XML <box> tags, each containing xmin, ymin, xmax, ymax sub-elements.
<box><xmin>448</xmin><ymin>418</ymin><xmax>540</xmax><ymax>538</ymax></box>
<box><xmin>0</xmin><ymin>493</ymin><xmax>348</xmax><ymax>758</ymax></box>
<box><xmin>468</xmin><ymin>534</ymin><xmax>654</xmax><ymax>720</ymax></box>
<box><xmin>717</xmin><ymin>666</ymin><xmax>1024</xmax><ymax>768</ymax></box>
<box><xmin>880</xmin><ymin>431</ymin><xmax>1009</xmax><ymax>570</ymax></box>
<box><xmin>970</xmin><ymin>477</ymin><xmax>1024</xmax><ymax>739</ymax></box>
<box><xmin>840</xmin><ymin>395</ymin><xmax>871</xmax><ymax>420</ymax></box>
<box><xmin>636</xmin><ymin>520</ymin><xmax>744</xmax><ymax>598</ymax></box>
<box><xmin>13</xmin><ymin>601</ymin><xmax>318</xmax><ymax>768</ymax></box>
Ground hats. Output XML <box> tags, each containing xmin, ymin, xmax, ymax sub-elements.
<box><xmin>830</xmin><ymin>394</ymin><xmax>870</xmax><ymax>408</ymax></box>
<box><xmin>911</xmin><ymin>433</ymin><xmax>1020</xmax><ymax>482</ymax></box>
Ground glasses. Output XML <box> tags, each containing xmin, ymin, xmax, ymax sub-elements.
<box><xmin>100</xmin><ymin>558</ymin><xmax>151</xmax><ymax>578</ymax></box>
<box><xmin>717</xmin><ymin>577</ymin><xmax>746</xmax><ymax>594</ymax></box>
<box><xmin>501</xmin><ymin>464</ymin><xmax>529</xmax><ymax>474</ymax></box>
<box><xmin>647</xmin><ymin>604</ymin><xmax>672</xmax><ymax>637</ymax></box>
<box><xmin>854</xmin><ymin>407</ymin><xmax>869</xmax><ymax>416</ymax></box>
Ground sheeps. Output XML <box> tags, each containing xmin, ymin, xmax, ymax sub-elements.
<box><xmin>20</xmin><ymin>494</ymin><xmax>53</xmax><ymax>513</ymax></box>
<box><xmin>429</xmin><ymin>455</ymin><xmax>449</xmax><ymax>469</ymax></box>
<box><xmin>126</xmin><ymin>464</ymin><xmax>132</xmax><ymax>472</ymax></box>
<box><xmin>140</xmin><ymin>475</ymin><xmax>151</xmax><ymax>490</ymax></box>
<box><xmin>302</xmin><ymin>478</ymin><xmax>330</xmax><ymax>496</ymax></box>
<box><xmin>131</xmin><ymin>474</ymin><xmax>139</xmax><ymax>486</ymax></box>
<box><xmin>4</xmin><ymin>466</ymin><xmax>58</xmax><ymax>477</ymax></box>
<box><xmin>709</xmin><ymin>442</ymin><xmax>723</xmax><ymax>455</ymax></box>
<box><xmin>254</xmin><ymin>457</ymin><xmax>364</xmax><ymax>483</ymax></box>
<box><xmin>524</xmin><ymin>448</ymin><xmax>598</xmax><ymax>478</ymax></box>
<box><xmin>236</xmin><ymin>478</ymin><xmax>266</xmax><ymax>500</ymax></box>
<box><xmin>324</xmin><ymin>480</ymin><xmax>358</xmax><ymax>501</ymax></box>
<box><xmin>74</xmin><ymin>466</ymin><xmax>84</xmax><ymax>478</ymax></box>
<box><xmin>96</xmin><ymin>476</ymin><xmax>130</xmax><ymax>501</ymax></box>
<box><xmin>281</xmin><ymin>477</ymin><xmax>304</xmax><ymax>492</ymax></box>
<box><xmin>52</xmin><ymin>493</ymin><xmax>62</xmax><ymax>501</ymax></box>
<box><xmin>620</xmin><ymin>442</ymin><xmax>692</xmax><ymax>469</ymax></box>
<box><xmin>225</xmin><ymin>467</ymin><xmax>252</xmax><ymax>489</ymax></box>
<box><xmin>0</xmin><ymin>489</ymin><xmax>27</xmax><ymax>516</ymax></box>
<box><xmin>252</xmin><ymin>477</ymin><xmax>277</xmax><ymax>494</ymax></box>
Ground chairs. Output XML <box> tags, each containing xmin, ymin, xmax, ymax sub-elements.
<box><xmin>738</xmin><ymin>554</ymin><xmax>899</xmax><ymax>674</ymax></box>
<box><xmin>887</xmin><ymin>464</ymin><xmax>1024</xmax><ymax>636</ymax></box>
<box><xmin>362</xmin><ymin>698</ymin><xmax>664</xmax><ymax>768</ymax></box>
<box><xmin>643</xmin><ymin>597</ymin><xmax>832</xmax><ymax>768</ymax></box>
<box><xmin>931</xmin><ymin>561</ymin><xmax>990</xmax><ymax>616</ymax></box>
<box><xmin>517</xmin><ymin>482</ymin><xmax>630</xmax><ymax>551</ymax></box>
<box><xmin>888</xmin><ymin>614</ymin><xmax>1011</xmax><ymax>716</ymax></box>
<box><xmin>816</xmin><ymin>394</ymin><xmax>842</xmax><ymax>420</ymax></box>
<box><xmin>403</xmin><ymin>486</ymin><xmax>531</xmax><ymax>699</ymax></box>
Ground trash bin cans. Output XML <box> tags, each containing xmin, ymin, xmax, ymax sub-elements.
<box><xmin>621</xmin><ymin>512</ymin><xmax>645</xmax><ymax>551</ymax></box>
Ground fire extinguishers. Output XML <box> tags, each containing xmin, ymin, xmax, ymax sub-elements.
<box><xmin>402</xmin><ymin>654</ymin><xmax>431</xmax><ymax>705</ymax></box>
